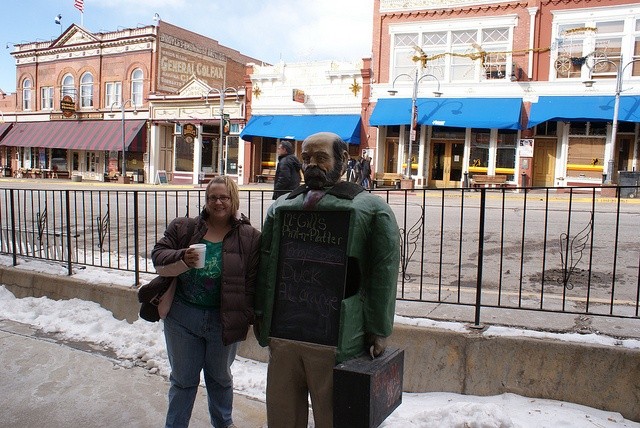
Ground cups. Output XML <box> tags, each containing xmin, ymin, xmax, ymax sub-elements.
<box><xmin>190</xmin><ymin>244</ymin><xmax>207</xmax><ymax>268</ymax></box>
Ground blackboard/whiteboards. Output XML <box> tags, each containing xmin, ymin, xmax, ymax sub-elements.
<box><xmin>270</xmin><ymin>210</ymin><xmax>350</xmax><ymax>347</ymax></box>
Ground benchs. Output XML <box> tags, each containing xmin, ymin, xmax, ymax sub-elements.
<box><xmin>472</xmin><ymin>174</ymin><xmax>506</xmax><ymax>191</ymax></box>
<box><xmin>256</xmin><ymin>169</ymin><xmax>276</xmax><ymax>183</ymax></box>
<box><xmin>371</xmin><ymin>172</ymin><xmax>403</xmax><ymax>190</ymax></box>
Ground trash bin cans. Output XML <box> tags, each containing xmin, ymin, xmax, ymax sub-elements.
<box><xmin>133</xmin><ymin>168</ymin><xmax>144</xmax><ymax>184</ymax></box>
<box><xmin>619</xmin><ymin>172</ymin><xmax>640</xmax><ymax>199</ymax></box>
<box><xmin>2</xmin><ymin>168</ymin><xmax>11</xmax><ymax>177</ymax></box>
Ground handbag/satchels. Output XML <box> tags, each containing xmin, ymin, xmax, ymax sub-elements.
<box><xmin>137</xmin><ymin>275</ymin><xmax>173</xmax><ymax>322</ymax></box>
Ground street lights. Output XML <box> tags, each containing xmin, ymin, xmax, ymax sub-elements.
<box><xmin>582</xmin><ymin>54</ymin><xmax>640</xmax><ymax>198</ymax></box>
<box><xmin>387</xmin><ymin>68</ymin><xmax>443</xmax><ymax>189</ymax></box>
<box><xmin>204</xmin><ymin>87</ymin><xmax>241</xmax><ymax>175</ymax></box>
<box><xmin>108</xmin><ymin>100</ymin><xmax>138</xmax><ymax>183</ymax></box>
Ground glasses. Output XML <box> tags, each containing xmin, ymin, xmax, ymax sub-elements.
<box><xmin>207</xmin><ymin>195</ymin><xmax>232</xmax><ymax>201</ymax></box>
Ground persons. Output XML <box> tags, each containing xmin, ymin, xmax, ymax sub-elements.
<box><xmin>151</xmin><ymin>175</ymin><xmax>261</xmax><ymax>426</ymax></box>
<box><xmin>253</xmin><ymin>132</ymin><xmax>400</xmax><ymax>428</ymax></box>
<box><xmin>272</xmin><ymin>141</ymin><xmax>302</xmax><ymax>200</ymax></box>
<box><xmin>346</xmin><ymin>154</ymin><xmax>372</xmax><ymax>189</ymax></box>
